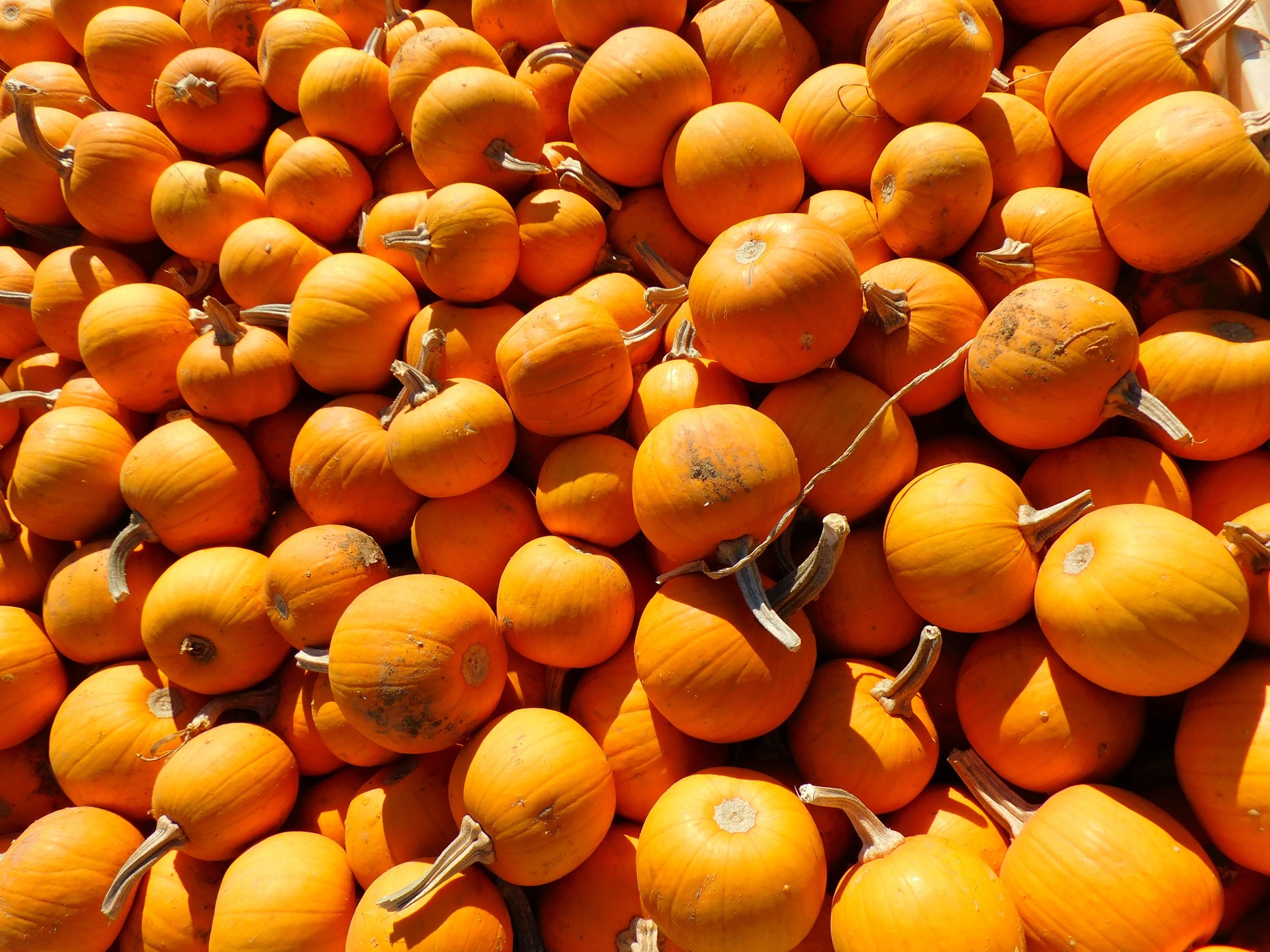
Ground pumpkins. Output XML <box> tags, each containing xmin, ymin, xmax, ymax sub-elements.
<box><xmin>0</xmin><ymin>0</ymin><xmax>1270</xmax><ymax>952</ymax></box>
